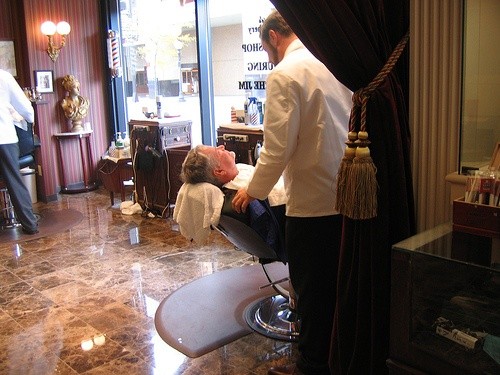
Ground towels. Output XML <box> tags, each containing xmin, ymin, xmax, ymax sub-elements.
<box><xmin>222</xmin><ymin>163</ymin><xmax>289</xmax><ymax>208</ymax></box>
<box><xmin>172</xmin><ymin>181</ymin><xmax>225</xmax><ymax>243</ymax></box>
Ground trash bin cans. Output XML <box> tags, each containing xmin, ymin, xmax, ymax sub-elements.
<box><xmin>20</xmin><ymin>168</ymin><xmax>38</xmax><ymax>204</ymax></box>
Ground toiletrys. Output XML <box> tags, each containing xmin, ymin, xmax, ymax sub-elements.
<box><xmin>115</xmin><ymin>132</ymin><xmax>130</xmax><ymax>147</ymax></box>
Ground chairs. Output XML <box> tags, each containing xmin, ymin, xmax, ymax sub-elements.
<box><xmin>0</xmin><ymin>119</ymin><xmax>41</xmax><ymax>229</ymax></box>
<box><xmin>171</xmin><ymin>182</ymin><xmax>301</xmax><ymax>343</ymax></box>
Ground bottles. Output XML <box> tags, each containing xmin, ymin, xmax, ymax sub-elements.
<box><xmin>156</xmin><ymin>95</ymin><xmax>164</xmax><ymax>119</ymax></box>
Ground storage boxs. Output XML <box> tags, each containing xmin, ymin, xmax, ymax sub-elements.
<box><xmin>452</xmin><ymin>196</ymin><xmax>500</xmax><ymax>240</ymax></box>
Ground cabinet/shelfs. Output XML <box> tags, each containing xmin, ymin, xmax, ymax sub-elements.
<box><xmin>128</xmin><ymin>119</ymin><xmax>191</xmax><ymax>218</ymax></box>
<box><xmin>101</xmin><ymin>156</ymin><xmax>134</xmax><ymax>205</ymax></box>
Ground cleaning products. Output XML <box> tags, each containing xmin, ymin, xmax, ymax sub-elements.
<box><xmin>247</xmin><ymin>97</ymin><xmax>258</xmax><ymax>126</ymax></box>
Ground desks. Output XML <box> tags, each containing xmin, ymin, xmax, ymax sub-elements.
<box><xmin>52</xmin><ymin>130</ymin><xmax>98</xmax><ymax>194</ymax></box>
<box><xmin>216</xmin><ymin>126</ymin><xmax>264</xmax><ymax>168</ymax></box>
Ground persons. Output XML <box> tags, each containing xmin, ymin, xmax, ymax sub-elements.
<box><xmin>181</xmin><ymin>145</ymin><xmax>288</xmax><ymax>207</ymax></box>
<box><xmin>232</xmin><ymin>11</ymin><xmax>354</xmax><ymax>375</ymax></box>
<box><xmin>60</xmin><ymin>75</ymin><xmax>89</xmax><ymax>132</ymax></box>
<box><xmin>0</xmin><ymin>70</ymin><xmax>40</xmax><ymax>233</ymax></box>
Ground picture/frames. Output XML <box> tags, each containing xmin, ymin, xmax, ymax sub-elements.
<box><xmin>34</xmin><ymin>69</ymin><xmax>55</xmax><ymax>94</ymax></box>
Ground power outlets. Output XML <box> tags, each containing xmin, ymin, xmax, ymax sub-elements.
<box><xmin>222</xmin><ymin>134</ymin><xmax>248</xmax><ymax>142</ymax></box>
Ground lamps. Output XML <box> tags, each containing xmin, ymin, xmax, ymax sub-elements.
<box><xmin>40</xmin><ymin>21</ymin><xmax>70</xmax><ymax>63</ymax></box>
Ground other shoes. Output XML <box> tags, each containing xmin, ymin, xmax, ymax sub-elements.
<box><xmin>268</xmin><ymin>364</ymin><xmax>307</xmax><ymax>375</ymax></box>
<box><xmin>22</xmin><ymin>227</ymin><xmax>39</xmax><ymax>235</ymax></box>
